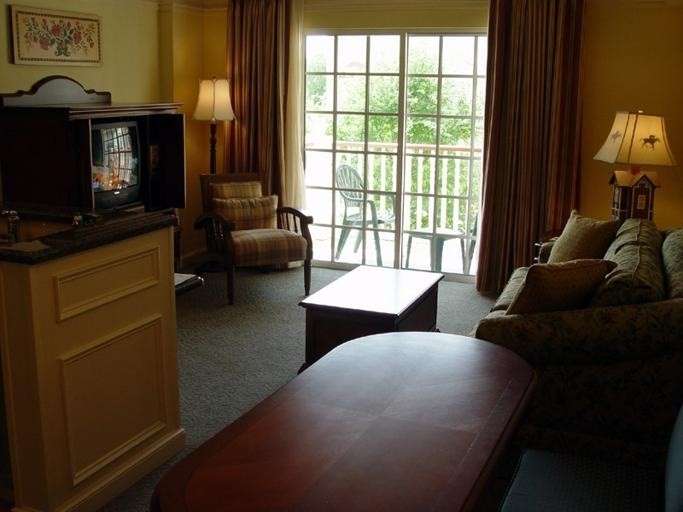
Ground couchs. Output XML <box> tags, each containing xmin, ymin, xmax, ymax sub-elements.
<box><xmin>475</xmin><ymin>227</ymin><xmax>683</xmax><ymax>470</ymax></box>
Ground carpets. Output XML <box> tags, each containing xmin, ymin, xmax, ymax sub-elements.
<box><xmin>100</xmin><ymin>269</ymin><xmax>495</xmax><ymax>511</ymax></box>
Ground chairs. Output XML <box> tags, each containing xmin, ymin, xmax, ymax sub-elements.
<box><xmin>200</xmin><ymin>174</ymin><xmax>313</xmax><ymax>304</ymax></box>
<box><xmin>464</xmin><ymin>212</ymin><xmax>478</xmax><ymax>274</ymax></box>
<box><xmin>336</xmin><ymin>165</ymin><xmax>396</xmax><ymax>266</ymax></box>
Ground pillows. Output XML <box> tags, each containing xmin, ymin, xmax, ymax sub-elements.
<box><xmin>547</xmin><ymin>209</ymin><xmax>621</xmax><ymax>265</ymax></box>
<box><xmin>505</xmin><ymin>258</ymin><xmax>617</xmax><ymax>316</ymax></box>
<box><xmin>211</xmin><ymin>194</ymin><xmax>279</xmax><ymax>231</ymax></box>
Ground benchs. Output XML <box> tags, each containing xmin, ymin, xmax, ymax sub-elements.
<box><xmin>497</xmin><ymin>402</ymin><xmax>682</xmax><ymax>512</ymax></box>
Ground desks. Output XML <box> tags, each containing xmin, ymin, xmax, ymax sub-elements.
<box><xmin>150</xmin><ymin>331</ymin><xmax>539</xmax><ymax>512</ymax></box>
<box><xmin>404</xmin><ymin>226</ymin><xmax>464</xmax><ymax>274</ymax></box>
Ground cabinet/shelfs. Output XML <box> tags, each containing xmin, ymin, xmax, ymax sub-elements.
<box><xmin>0</xmin><ymin>75</ymin><xmax>186</xmax><ymax>221</ymax></box>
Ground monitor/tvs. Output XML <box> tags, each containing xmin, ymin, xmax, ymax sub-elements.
<box><xmin>87</xmin><ymin>120</ymin><xmax>148</xmax><ymax>219</ymax></box>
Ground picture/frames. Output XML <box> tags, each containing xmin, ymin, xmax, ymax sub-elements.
<box><xmin>11</xmin><ymin>5</ymin><xmax>105</xmax><ymax>67</ymax></box>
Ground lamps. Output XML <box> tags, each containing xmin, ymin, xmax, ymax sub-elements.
<box><xmin>191</xmin><ymin>76</ymin><xmax>236</xmax><ymax>274</ymax></box>
<box><xmin>593</xmin><ymin>110</ymin><xmax>678</xmax><ymax>220</ymax></box>
